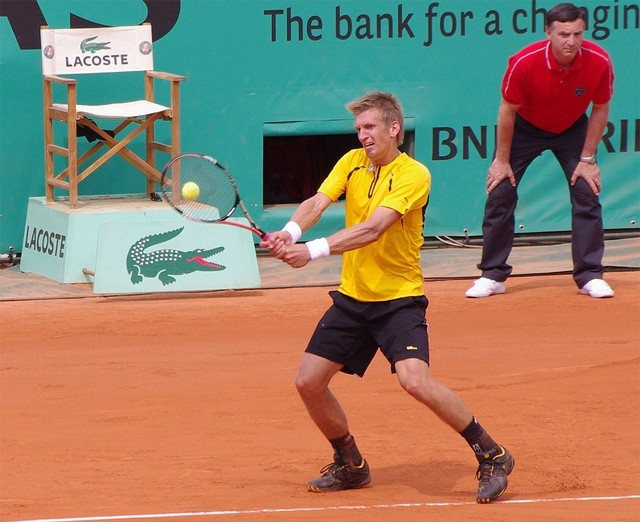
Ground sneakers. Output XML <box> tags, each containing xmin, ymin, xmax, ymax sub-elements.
<box><xmin>464</xmin><ymin>277</ymin><xmax>506</xmax><ymax>299</ymax></box>
<box><xmin>578</xmin><ymin>279</ymin><xmax>614</xmax><ymax>298</ymax></box>
<box><xmin>475</xmin><ymin>445</ymin><xmax>514</xmax><ymax>503</ymax></box>
<box><xmin>308</xmin><ymin>456</ymin><xmax>371</xmax><ymax>493</ymax></box>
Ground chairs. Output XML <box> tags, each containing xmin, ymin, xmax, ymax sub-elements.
<box><xmin>40</xmin><ymin>23</ymin><xmax>187</xmax><ymax>209</ymax></box>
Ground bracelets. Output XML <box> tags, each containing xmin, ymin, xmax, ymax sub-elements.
<box><xmin>304</xmin><ymin>237</ymin><xmax>332</xmax><ymax>262</ymax></box>
<box><xmin>280</xmin><ymin>221</ymin><xmax>303</xmax><ymax>244</ymax></box>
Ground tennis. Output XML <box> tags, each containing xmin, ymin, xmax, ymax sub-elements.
<box><xmin>181</xmin><ymin>182</ymin><xmax>201</xmax><ymax>201</ymax></box>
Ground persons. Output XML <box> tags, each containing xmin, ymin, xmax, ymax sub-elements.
<box><xmin>260</xmin><ymin>91</ymin><xmax>516</xmax><ymax>504</ymax></box>
<box><xmin>465</xmin><ymin>3</ymin><xmax>614</xmax><ymax>298</ymax></box>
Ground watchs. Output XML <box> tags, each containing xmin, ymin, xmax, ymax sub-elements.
<box><xmin>580</xmin><ymin>155</ymin><xmax>599</xmax><ymax>166</ymax></box>
